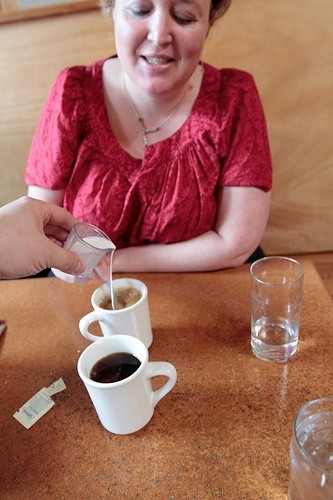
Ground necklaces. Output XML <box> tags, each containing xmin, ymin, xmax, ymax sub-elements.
<box><xmin>121</xmin><ymin>71</ymin><xmax>196</xmax><ymax>147</ymax></box>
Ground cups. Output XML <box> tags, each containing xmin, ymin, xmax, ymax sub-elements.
<box><xmin>51</xmin><ymin>222</ymin><xmax>116</xmax><ymax>283</ymax></box>
<box><xmin>77</xmin><ymin>334</ymin><xmax>177</xmax><ymax>435</ymax></box>
<box><xmin>250</xmin><ymin>256</ymin><xmax>304</xmax><ymax>363</ymax></box>
<box><xmin>287</xmin><ymin>398</ymin><xmax>333</xmax><ymax>500</ymax></box>
<box><xmin>79</xmin><ymin>278</ymin><xmax>154</xmax><ymax>349</ymax></box>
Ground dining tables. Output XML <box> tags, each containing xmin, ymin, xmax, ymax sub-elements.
<box><xmin>0</xmin><ymin>257</ymin><xmax>332</xmax><ymax>500</ymax></box>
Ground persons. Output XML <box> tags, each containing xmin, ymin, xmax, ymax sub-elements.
<box><xmin>25</xmin><ymin>0</ymin><xmax>273</xmax><ymax>279</ymax></box>
<box><xmin>0</xmin><ymin>196</ymin><xmax>85</xmax><ymax>280</ymax></box>
